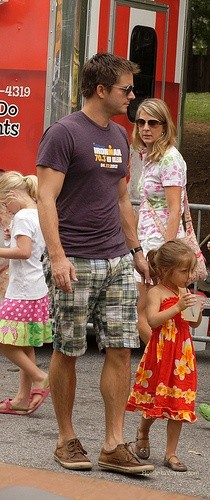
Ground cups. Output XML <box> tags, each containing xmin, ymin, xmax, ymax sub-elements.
<box><xmin>184</xmin><ymin>295</ymin><xmax>204</xmax><ymax>323</ymax></box>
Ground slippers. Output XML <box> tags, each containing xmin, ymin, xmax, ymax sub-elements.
<box><xmin>27</xmin><ymin>384</ymin><xmax>51</xmax><ymax>414</ymax></box>
<box><xmin>0</xmin><ymin>398</ymin><xmax>30</xmax><ymax>415</ymax></box>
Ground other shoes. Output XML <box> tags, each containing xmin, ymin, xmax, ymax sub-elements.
<box><xmin>135</xmin><ymin>427</ymin><xmax>151</xmax><ymax>459</ymax></box>
<box><xmin>164</xmin><ymin>453</ymin><xmax>187</xmax><ymax>471</ymax></box>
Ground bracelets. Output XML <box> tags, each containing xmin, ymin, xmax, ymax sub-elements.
<box><xmin>130</xmin><ymin>246</ymin><xmax>142</xmax><ymax>255</ymax></box>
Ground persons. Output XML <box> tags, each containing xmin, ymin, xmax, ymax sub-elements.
<box><xmin>126</xmin><ymin>239</ymin><xmax>205</xmax><ymax>472</ymax></box>
<box><xmin>35</xmin><ymin>51</ymin><xmax>156</xmax><ymax>476</ymax></box>
<box><xmin>0</xmin><ymin>170</ymin><xmax>53</xmax><ymax>416</ymax></box>
<box><xmin>126</xmin><ymin>98</ymin><xmax>188</xmax><ymax>348</ymax></box>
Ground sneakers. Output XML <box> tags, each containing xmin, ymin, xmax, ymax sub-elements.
<box><xmin>54</xmin><ymin>439</ymin><xmax>92</xmax><ymax>470</ymax></box>
<box><xmin>98</xmin><ymin>441</ymin><xmax>155</xmax><ymax>474</ymax></box>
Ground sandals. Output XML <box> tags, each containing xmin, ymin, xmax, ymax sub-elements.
<box><xmin>198</xmin><ymin>403</ymin><xmax>210</xmax><ymax>422</ymax></box>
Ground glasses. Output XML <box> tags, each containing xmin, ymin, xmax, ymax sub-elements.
<box><xmin>113</xmin><ymin>85</ymin><xmax>136</xmax><ymax>97</ymax></box>
<box><xmin>135</xmin><ymin>119</ymin><xmax>161</xmax><ymax>128</ymax></box>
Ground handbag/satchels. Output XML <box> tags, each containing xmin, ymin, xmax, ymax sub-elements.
<box><xmin>175</xmin><ymin>229</ymin><xmax>208</xmax><ymax>287</ymax></box>
